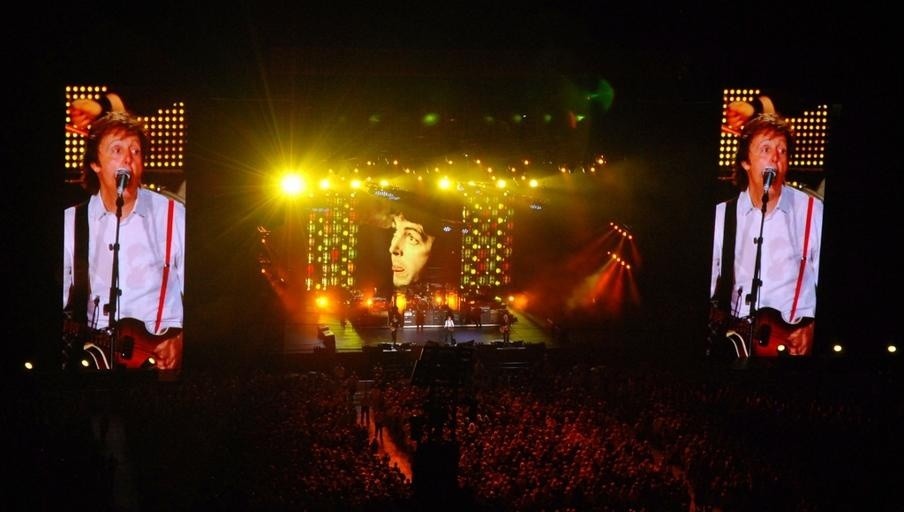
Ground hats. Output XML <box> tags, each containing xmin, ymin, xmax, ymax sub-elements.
<box><xmin>349</xmin><ymin>185</ymin><xmax>462</xmax><ymax>237</ymax></box>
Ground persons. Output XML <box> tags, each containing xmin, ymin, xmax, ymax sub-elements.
<box><xmin>338</xmin><ymin>284</ymin><xmax>512</xmax><ymax>344</ymax></box>
<box><xmin>708</xmin><ymin>112</ymin><xmax>824</xmax><ymax>357</ymax></box>
<box><xmin>385</xmin><ymin>201</ymin><xmax>439</xmax><ymax>288</ymax></box>
<box><xmin>62</xmin><ymin>110</ymin><xmax>183</xmax><ymax>373</ymax></box>
<box><xmin>1</xmin><ymin>364</ymin><xmax>904</xmax><ymax>512</ymax></box>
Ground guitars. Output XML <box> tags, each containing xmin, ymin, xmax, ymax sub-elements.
<box><xmin>63</xmin><ymin>317</ymin><xmax>184</xmax><ymax>370</ymax></box>
<box><xmin>711</xmin><ymin>306</ymin><xmax>815</xmax><ymax>356</ymax></box>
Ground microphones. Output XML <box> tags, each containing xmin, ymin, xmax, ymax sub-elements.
<box><xmin>114</xmin><ymin>169</ymin><xmax>131</xmax><ymax>198</ymax></box>
<box><xmin>762</xmin><ymin>168</ymin><xmax>776</xmax><ymax>194</ymax></box>
